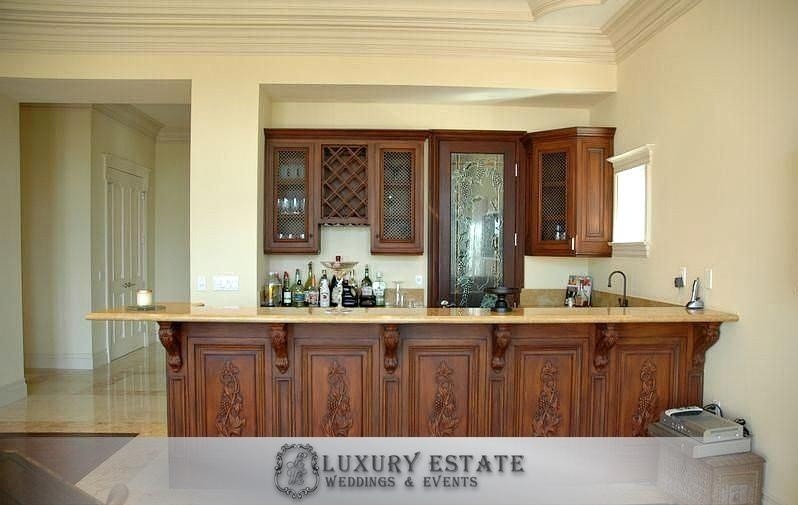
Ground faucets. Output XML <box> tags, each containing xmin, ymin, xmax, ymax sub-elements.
<box><xmin>607</xmin><ymin>270</ymin><xmax>628</xmax><ymax>307</ymax></box>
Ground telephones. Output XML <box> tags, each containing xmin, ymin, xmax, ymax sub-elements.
<box><xmin>686</xmin><ymin>278</ymin><xmax>704</xmax><ymax>309</ymax></box>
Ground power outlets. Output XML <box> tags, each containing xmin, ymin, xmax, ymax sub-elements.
<box><xmin>676</xmin><ymin>268</ymin><xmax>685</xmax><ymax>287</ymax></box>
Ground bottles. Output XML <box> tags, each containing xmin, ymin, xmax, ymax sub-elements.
<box><xmin>262</xmin><ymin>256</ymin><xmax>418</xmax><ymax>309</ymax></box>
<box><xmin>555</xmin><ymin>224</ymin><xmax>566</xmax><ymax>242</ymax></box>
<box><xmin>565</xmin><ymin>279</ymin><xmax>587</xmax><ymax>307</ymax></box>
<box><xmin>136</xmin><ymin>289</ymin><xmax>152</xmax><ymax>306</ymax></box>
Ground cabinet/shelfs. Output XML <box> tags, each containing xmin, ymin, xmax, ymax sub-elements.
<box><xmin>267</xmin><ymin>138</ymin><xmax>314</xmax><ymax>248</ymax></box>
<box><xmin>575</xmin><ymin>137</ymin><xmax>614</xmax><ymax>252</ymax></box>
<box><xmin>530</xmin><ymin>137</ymin><xmax>576</xmax><ymax>253</ymax></box>
<box><xmin>374</xmin><ymin>140</ymin><xmax>419</xmax><ymax>248</ymax></box>
<box><xmin>314</xmin><ymin>138</ymin><xmax>374</xmax><ymax>226</ymax></box>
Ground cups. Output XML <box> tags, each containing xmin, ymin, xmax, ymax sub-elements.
<box><xmin>276</xmin><ymin>197</ymin><xmax>308</xmax><ymax>214</ymax></box>
<box><xmin>278</xmin><ymin>232</ymin><xmax>308</xmax><ymax>240</ymax></box>
